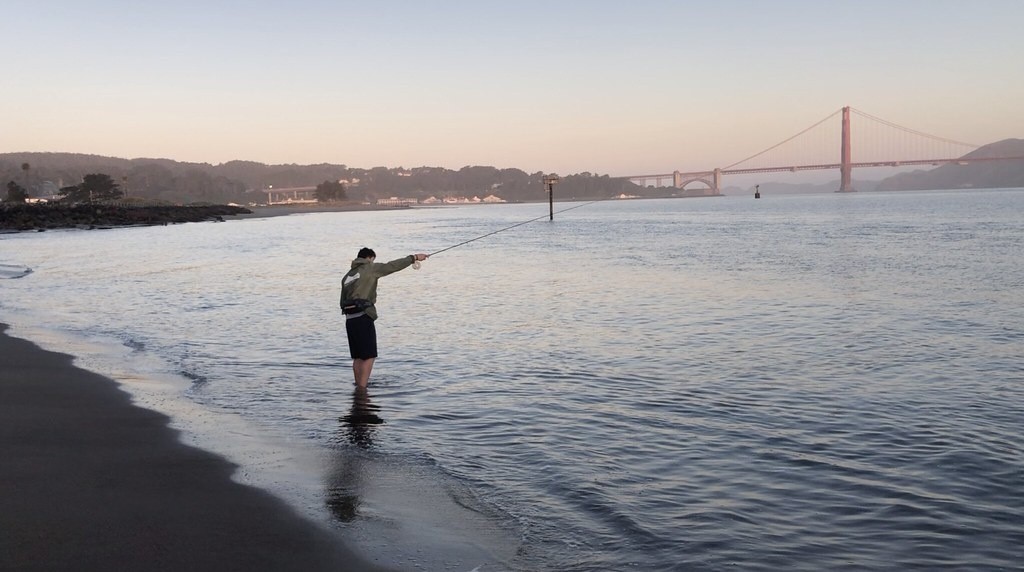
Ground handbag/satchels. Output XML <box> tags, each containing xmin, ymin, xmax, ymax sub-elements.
<box><xmin>341</xmin><ymin>299</ymin><xmax>374</xmax><ymax>315</ymax></box>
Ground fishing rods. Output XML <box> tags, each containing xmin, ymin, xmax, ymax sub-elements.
<box><xmin>412</xmin><ymin>201</ymin><xmax>595</xmax><ymax>269</ymax></box>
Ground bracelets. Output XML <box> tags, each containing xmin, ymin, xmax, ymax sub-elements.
<box><xmin>415</xmin><ymin>254</ymin><xmax>418</xmax><ymax>260</ymax></box>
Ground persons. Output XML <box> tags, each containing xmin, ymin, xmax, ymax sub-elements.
<box><xmin>339</xmin><ymin>247</ymin><xmax>429</xmax><ymax>387</ymax></box>
